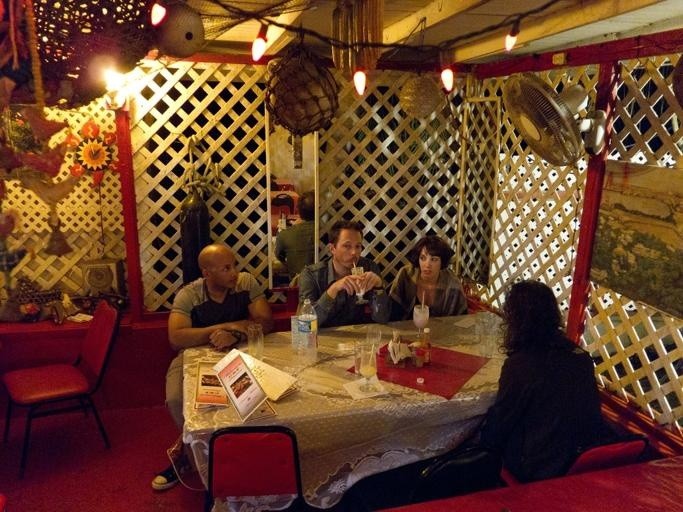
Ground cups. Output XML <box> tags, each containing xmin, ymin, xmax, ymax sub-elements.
<box><xmin>247</xmin><ymin>325</ymin><xmax>263</xmax><ymax>360</ymax></box>
<box><xmin>354</xmin><ymin>343</ymin><xmax>374</xmax><ymax>375</ymax></box>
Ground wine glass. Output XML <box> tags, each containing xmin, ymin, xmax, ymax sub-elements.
<box><xmin>360</xmin><ymin>347</ymin><xmax>376</xmax><ymax>393</ymax></box>
<box><xmin>413</xmin><ymin>305</ymin><xmax>429</xmax><ymax>339</ymax></box>
<box><xmin>351</xmin><ymin>267</ymin><xmax>369</xmax><ymax>304</ymax></box>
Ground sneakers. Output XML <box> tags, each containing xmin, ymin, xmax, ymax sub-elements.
<box><xmin>152</xmin><ymin>456</ymin><xmax>190</xmax><ymax>491</ymax></box>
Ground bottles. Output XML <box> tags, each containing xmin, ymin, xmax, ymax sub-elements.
<box><xmin>294</xmin><ymin>295</ymin><xmax>321</xmax><ymax>367</ymax></box>
<box><xmin>416</xmin><ymin>327</ymin><xmax>432</xmax><ymax>367</ymax></box>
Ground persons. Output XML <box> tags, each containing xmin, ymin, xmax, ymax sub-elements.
<box><xmin>481</xmin><ymin>279</ymin><xmax>601</xmax><ymax>480</ymax></box>
<box><xmin>274</xmin><ymin>190</ymin><xmax>315</xmax><ymax>287</ymax></box>
<box><xmin>295</xmin><ymin>220</ymin><xmax>391</xmax><ymax>328</ymax></box>
<box><xmin>151</xmin><ymin>243</ymin><xmax>276</xmax><ymax>491</ymax></box>
<box><xmin>387</xmin><ymin>235</ymin><xmax>468</xmax><ymax>322</ymax></box>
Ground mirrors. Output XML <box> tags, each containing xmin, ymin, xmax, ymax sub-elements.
<box><xmin>266</xmin><ymin>104</ymin><xmax>321</xmax><ymax>292</ymax></box>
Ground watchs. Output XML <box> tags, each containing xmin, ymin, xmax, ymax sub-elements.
<box><xmin>231</xmin><ymin>330</ymin><xmax>241</xmax><ymax>344</ymax></box>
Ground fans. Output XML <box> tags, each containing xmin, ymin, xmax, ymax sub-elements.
<box><xmin>501</xmin><ymin>72</ymin><xmax>589</xmax><ymax>168</ymax></box>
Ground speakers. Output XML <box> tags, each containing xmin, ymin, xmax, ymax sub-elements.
<box><xmin>82</xmin><ymin>260</ymin><xmax>122</xmax><ymax>293</ymax></box>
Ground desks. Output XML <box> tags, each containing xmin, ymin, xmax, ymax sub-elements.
<box><xmin>372</xmin><ymin>455</ymin><xmax>683</xmax><ymax>512</ymax></box>
<box><xmin>271</xmin><ymin>179</ymin><xmax>302</xmax><ymax>276</ymax></box>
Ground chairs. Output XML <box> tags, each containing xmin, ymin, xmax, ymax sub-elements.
<box><xmin>208</xmin><ymin>425</ymin><xmax>308</xmax><ymax>512</ymax></box>
<box><xmin>0</xmin><ymin>297</ymin><xmax>122</xmax><ymax>481</ymax></box>
<box><xmin>566</xmin><ymin>436</ymin><xmax>650</xmax><ymax>472</ymax></box>
